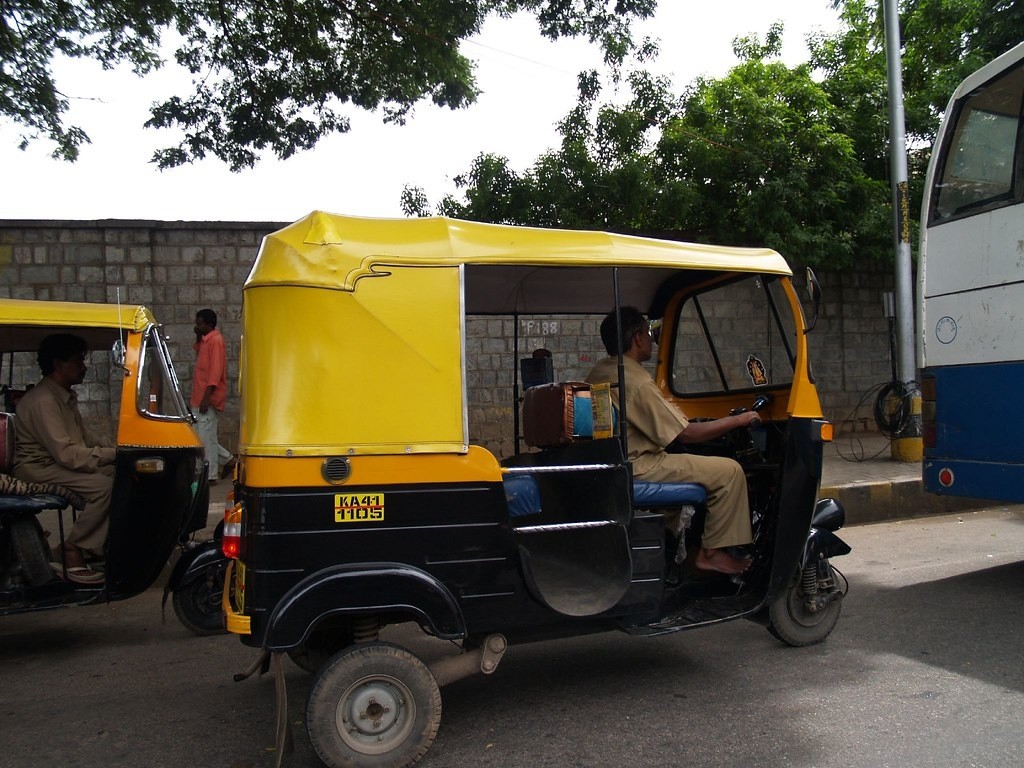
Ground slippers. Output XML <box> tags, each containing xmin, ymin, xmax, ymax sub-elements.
<box><xmin>50</xmin><ymin>555</ymin><xmax>106</xmax><ymax>580</ymax></box>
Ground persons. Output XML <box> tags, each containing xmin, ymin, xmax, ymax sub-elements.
<box><xmin>584</xmin><ymin>303</ymin><xmax>763</xmax><ymax>578</ymax></box>
<box><xmin>9</xmin><ymin>331</ymin><xmax>118</xmax><ymax>582</ymax></box>
<box><xmin>188</xmin><ymin>308</ymin><xmax>239</xmax><ymax>487</ymax></box>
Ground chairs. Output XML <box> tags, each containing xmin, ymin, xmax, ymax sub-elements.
<box><xmin>625</xmin><ymin>481</ymin><xmax>707</xmax><ymax>511</ymax></box>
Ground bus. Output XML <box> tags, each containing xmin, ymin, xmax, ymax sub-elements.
<box><xmin>920</xmin><ymin>31</ymin><xmax>1022</xmax><ymax>510</ymax></box>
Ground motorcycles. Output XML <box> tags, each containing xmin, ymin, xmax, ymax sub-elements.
<box><xmin>224</xmin><ymin>209</ymin><xmax>861</xmax><ymax>768</ymax></box>
<box><xmin>0</xmin><ymin>290</ymin><xmax>244</xmax><ymax>641</ymax></box>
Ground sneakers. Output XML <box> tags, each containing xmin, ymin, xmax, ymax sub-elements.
<box><xmin>208</xmin><ymin>479</ymin><xmax>217</xmax><ymax>487</ymax></box>
<box><xmin>221</xmin><ymin>457</ymin><xmax>238</xmax><ymax>480</ymax></box>
